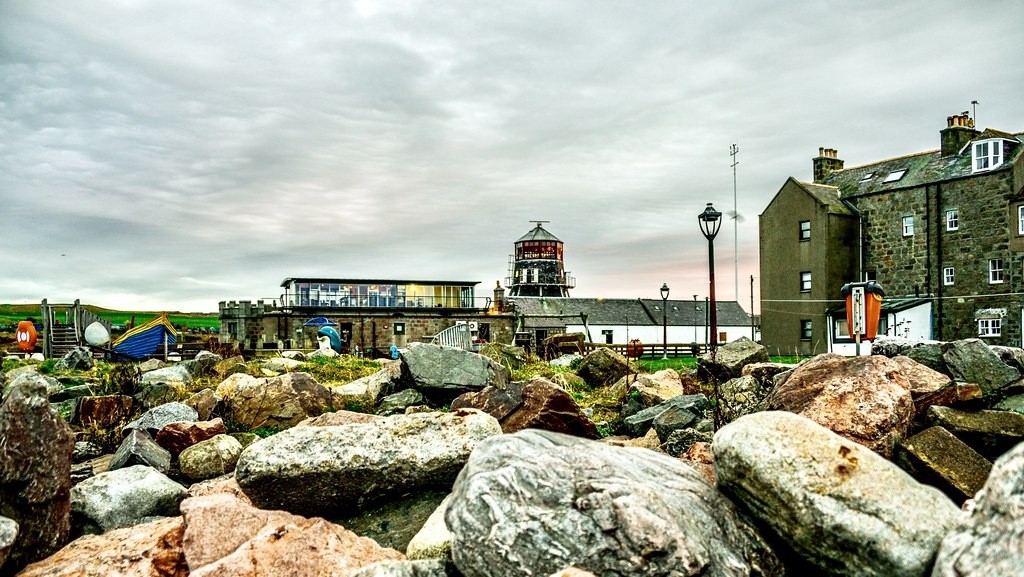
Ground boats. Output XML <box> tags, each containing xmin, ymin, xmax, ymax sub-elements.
<box><xmin>109</xmin><ymin>310</ymin><xmax>179</xmax><ymax>361</ymax></box>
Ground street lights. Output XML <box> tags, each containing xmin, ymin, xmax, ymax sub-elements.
<box><xmin>660</xmin><ymin>281</ymin><xmax>670</xmax><ymax>360</ymax></box>
<box><xmin>698</xmin><ymin>203</ymin><xmax>723</xmax><ymax>349</ymax></box>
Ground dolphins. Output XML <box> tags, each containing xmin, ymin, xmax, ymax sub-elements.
<box><xmin>317</xmin><ymin>326</ymin><xmax>341</xmax><ymax>357</ymax></box>
